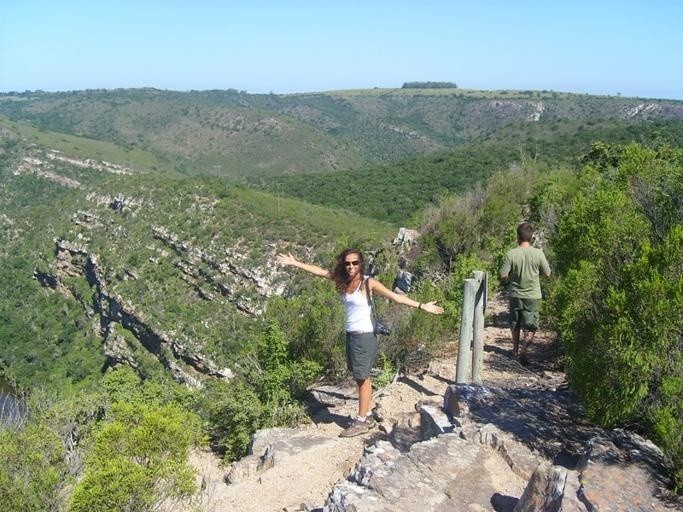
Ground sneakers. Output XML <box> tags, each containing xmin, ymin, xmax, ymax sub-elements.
<box><xmin>341</xmin><ymin>418</ymin><xmax>369</xmax><ymax>437</ymax></box>
<box><xmin>348</xmin><ymin>414</ymin><xmax>375</xmax><ymax>428</ymax></box>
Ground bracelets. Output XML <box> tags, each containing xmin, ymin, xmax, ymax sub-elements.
<box><xmin>418</xmin><ymin>302</ymin><xmax>422</xmax><ymax>310</ymax></box>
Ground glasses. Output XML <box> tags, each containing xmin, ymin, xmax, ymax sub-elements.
<box><xmin>344</xmin><ymin>261</ymin><xmax>361</xmax><ymax>267</ymax></box>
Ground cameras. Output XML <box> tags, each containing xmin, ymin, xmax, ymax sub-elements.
<box><xmin>374</xmin><ymin>322</ymin><xmax>391</xmax><ymax>337</ymax></box>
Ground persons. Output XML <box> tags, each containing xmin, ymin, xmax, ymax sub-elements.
<box><xmin>274</xmin><ymin>248</ymin><xmax>444</xmax><ymax>438</ymax></box>
<box><xmin>497</xmin><ymin>222</ymin><xmax>552</xmax><ymax>365</ymax></box>
